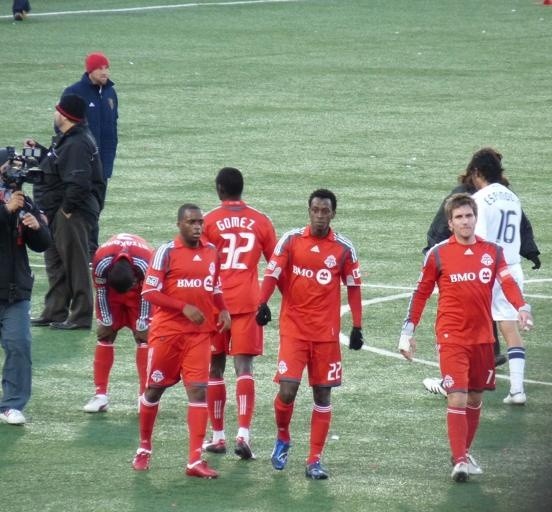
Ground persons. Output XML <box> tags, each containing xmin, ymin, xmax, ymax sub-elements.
<box><xmin>29</xmin><ymin>96</ymin><xmax>109</xmax><ymax>332</ymax></box>
<box><xmin>255</xmin><ymin>187</ymin><xmax>367</xmax><ymax>480</ymax></box>
<box><xmin>200</xmin><ymin>165</ymin><xmax>280</xmax><ymax>463</ymax></box>
<box><xmin>394</xmin><ymin>193</ymin><xmax>534</xmax><ymax>487</ymax></box>
<box><xmin>130</xmin><ymin>201</ymin><xmax>233</xmax><ymax>480</ymax></box>
<box><xmin>1</xmin><ymin>147</ymin><xmax>53</xmax><ymax>426</ymax></box>
<box><xmin>52</xmin><ymin>53</ymin><xmax>119</xmax><ymax>183</ymax></box>
<box><xmin>12</xmin><ymin>1</ymin><xmax>32</xmax><ymax>21</ymax></box>
<box><xmin>418</xmin><ymin>147</ymin><xmax>529</xmax><ymax>409</ymax></box>
<box><xmin>80</xmin><ymin>232</ymin><xmax>155</xmax><ymax>415</ymax></box>
<box><xmin>421</xmin><ymin>161</ymin><xmax>542</xmax><ymax>369</ymax></box>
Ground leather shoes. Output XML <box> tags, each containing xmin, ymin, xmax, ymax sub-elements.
<box><xmin>28</xmin><ymin>316</ymin><xmax>93</xmax><ymax>330</ymax></box>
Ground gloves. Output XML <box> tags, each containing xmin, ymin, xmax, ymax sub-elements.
<box><xmin>255</xmin><ymin>304</ymin><xmax>272</xmax><ymax>327</ymax></box>
<box><xmin>525</xmin><ymin>251</ymin><xmax>543</xmax><ymax>271</ymax></box>
<box><xmin>349</xmin><ymin>328</ymin><xmax>364</xmax><ymax>351</ymax></box>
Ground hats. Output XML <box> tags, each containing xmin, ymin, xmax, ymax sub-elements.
<box><xmin>85</xmin><ymin>53</ymin><xmax>109</xmax><ymax>74</ymax></box>
<box><xmin>55</xmin><ymin>95</ymin><xmax>86</xmax><ymax>123</ymax></box>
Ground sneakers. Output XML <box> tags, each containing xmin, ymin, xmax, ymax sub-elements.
<box><xmin>133</xmin><ymin>448</ymin><xmax>152</xmax><ymax>470</ymax></box>
<box><xmin>503</xmin><ymin>392</ymin><xmax>526</xmax><ymax>404</ymax></box>
<box><xmin>186</xmin><ymin>459</ymin><xmax>218</xmax><ymax>478</ymax></box>
<box><xmin>271</xmin><ymin>439</ymin><xmax>290</xmax><ymax>469</ymax></box>
<box><xmin>306</xmin><ymin>463</ymin><xmax>328</xmax><ymax>479</ymax></box>
<box><xmin>83</xmin><ymin>395</ymin><xmax>108</xmax><ymax>413</ymax></box>
<box><xmin>1</xmin><ymin>409</ymin><xmax>26</xmax><ymax>424</ymax></box>
<box><xmin>201</xmin><ymin>437</ymin><xmax>255</xmax><ymax>458</ymax></box>
<box><xmin>14</xmin><ymin>10</ymin><xmax>26</xmax><ymax>20</ymax></box>
<box><xmin>452</xmin><ymin>453</ymin><xmax>483</xmax><ymax>480</ymax></box>
<box><xmin>423</xmin><ymin>378</ymin><xmax>447</xmax><ymax>399</ymax></box>
